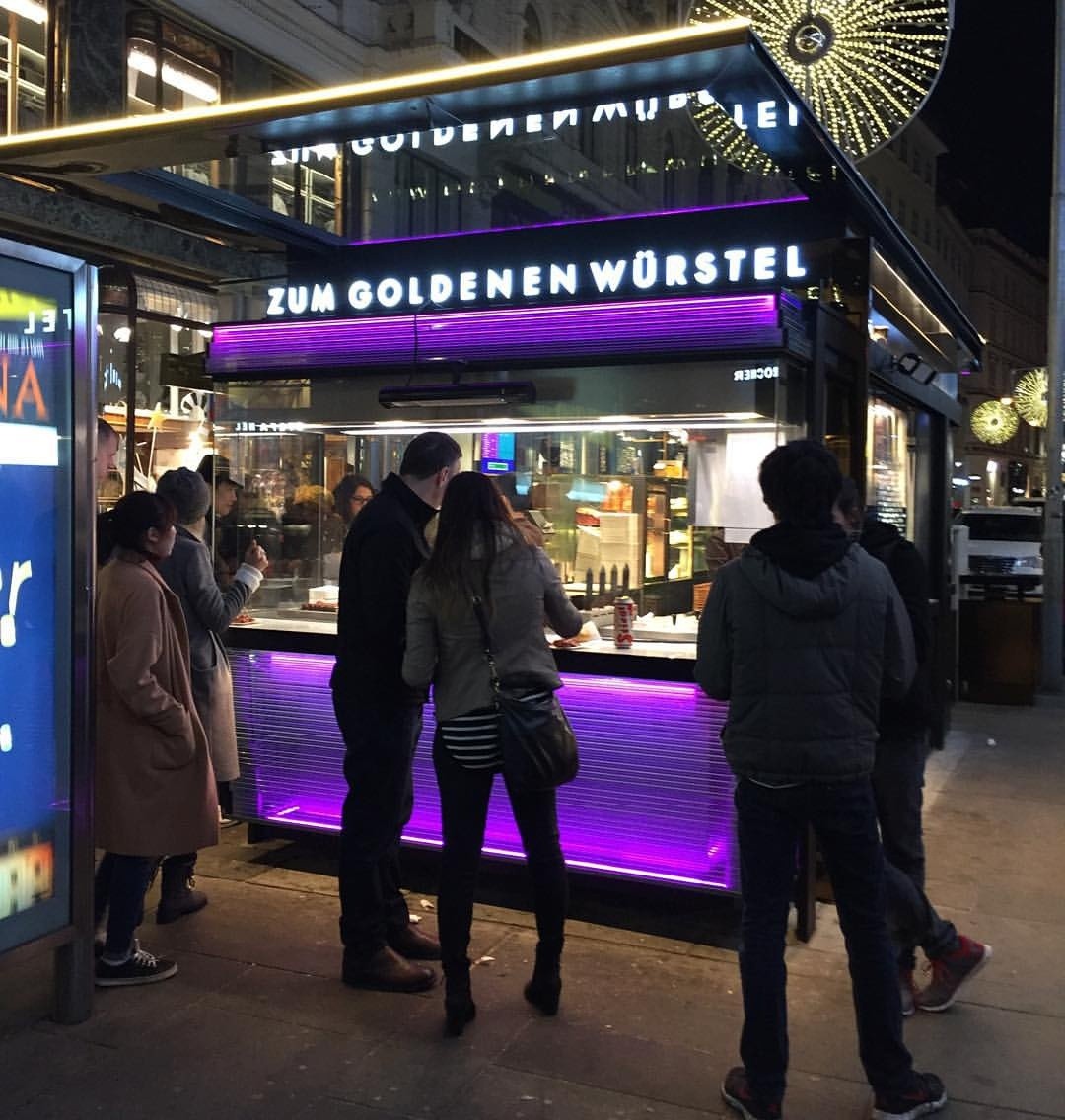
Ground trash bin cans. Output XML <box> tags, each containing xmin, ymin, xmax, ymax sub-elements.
<box><xmin>956</xmin><ymin>573</ymin><xmax>1045</xmax><ymax>706</ymax></box>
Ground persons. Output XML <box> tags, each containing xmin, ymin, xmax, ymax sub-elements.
<box><xmin>336</xmin><ymin>475</ymin><xmax>374</xmax><ymax>517</ymax></box>
<box><xmin>196</xmin><ymin>455</ymin><xmax>242</xmax><ymax>516</ymax></box>
<box><xmin>94</xmin><ymin>416</ymin><xmax>120</xmax><ymax>558</ymax></box>
<box><xmin>696</xmin><ymin>440</ymin><xmax>948</xmax><ymax>1119</ymax></box>
<box><xmin>158</xmin><ymin>468</ymin><xmax>270</xmax><ymax>782</ymax></box>
<box><xmin>89</xmin><ymin>491</ymin><xmax>219</xmax><ymax>984</ymax></box>
<box><xmin>832</xmin><ymin>484</ymin><xmax>992</xmax><ymax>1014</ymax></box>
<box><xmin>404</xmin><ymin>472</ymin><xmax>581</xmax><ymax>1016</ymax></box>
<box><xmin>330</xmin><ymin>432</ymin><xmax>462</xmax><ymax>993</ymax></box>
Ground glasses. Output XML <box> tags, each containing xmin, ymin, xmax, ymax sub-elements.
<box><xmin>351</xmin><ymin>494</ymin><xmax>373</xmax><ymax>505</ymax></box>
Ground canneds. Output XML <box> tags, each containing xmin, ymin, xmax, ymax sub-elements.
<box><xmin>614</xmin><ymin>598</ymin><xmax>634</xmax><ymax>650</ymax></box>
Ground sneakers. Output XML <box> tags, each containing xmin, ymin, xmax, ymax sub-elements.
<box><xmin>342</xmin><ymin>943</ymin><xmax>436</xmax><ymax>993</ymax></box>
<box><xmin>445</xmin><ymin>997</ymin><xmax>479</xmax><ymax>1037</ymax></box>
<box><xmin>873</xmin><ymin>1068</ymin><xmax>948</xmax><ymax>1120</ymax></box>
<box><xmin>721</xmin><ymin>1064</ymin><xmax>784</xmax><ymax>1120</ymax></box>
<box><xmin>912</xmin><ymin>934</ymin><xmax>993</xmax><ymax>1013</ymax></box>
<box><xmin>94</xmin><ymin>927</ymin><xmax>177</xmax><ymax>987</ymax></box>
<box><xmin>898</xmin><ymin>968</ymin><xmax>920</xmax><ymax>1015</ymax></box>
<box><xmin>522</xmin><ymin>976</ymin><xmax>558</xmax><ymax>1013</ymax></box>
<box><xmin>154</xmin><ymin>880</ymin><xmax>208</xmax><ymax>924</ymax></box>
<box><xmin>395</xmin><ymin>921</ymin><xmax>444</xmax><ymax>961</ymax></box>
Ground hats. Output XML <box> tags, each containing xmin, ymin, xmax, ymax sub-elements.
<box><xmin>291</xmin><ymin>482</ymin><xmax>337</xmax><ymax>515</ymax></box>
<box><xmin>195</xmin><ymin>453</ymin><xmax>244</xmax><ymax>489</ymax></box>
<box><xmin>156</xmin><ymin>466</ymin><xmax>211</xmax><ymax>524</ymax></box>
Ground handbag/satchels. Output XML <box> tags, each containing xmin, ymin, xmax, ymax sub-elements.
<box><xmin>490</xmin><ymin>679</ymin><xmax>579</xmax><ymax>788</ymax></box>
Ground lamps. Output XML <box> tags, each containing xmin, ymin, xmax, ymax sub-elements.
<box><xmin>379</xmin><ymin>359</ymin><xmax>535</xmax><ymax>411</ymax></box>
<box><xmin>893</xmin><ymin>352</ymin><xmax>937</xmax><ymax>386</ymax></box>
